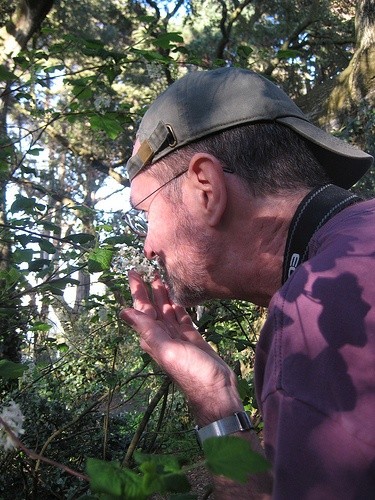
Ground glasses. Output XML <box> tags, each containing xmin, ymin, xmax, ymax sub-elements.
<box><xmin>121</xmin><ymin>167</ymin><xmax>234</xmax><ymax>237</ymax></box>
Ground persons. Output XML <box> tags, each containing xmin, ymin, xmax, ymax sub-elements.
<box><xmin>116</xmin><ymin>64</ymin><xmax>374</xmax><ymax>500</ymax></box>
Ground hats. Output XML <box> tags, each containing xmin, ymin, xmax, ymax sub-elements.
<box><xmin>127</xmin><ymin>67</ymin><xmax>374</xmax><ymax>189</ymax></box>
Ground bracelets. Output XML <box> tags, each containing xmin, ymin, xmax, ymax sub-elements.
<box><xmin>194</xmin><ymin>411</ymin><xmax>254</xmax><ymax>445</ymax></box>
